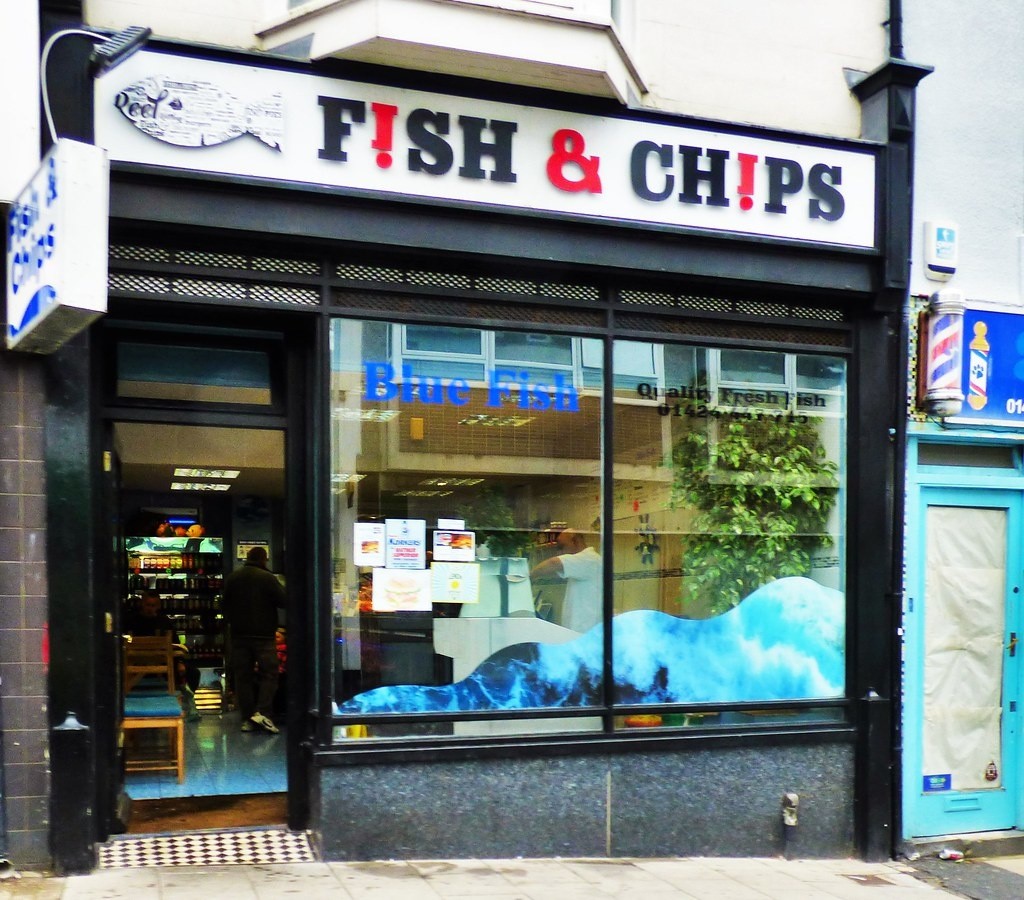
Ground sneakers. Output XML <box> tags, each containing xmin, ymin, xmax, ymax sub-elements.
<box><xmin>241</xmin><ymin>721</ymin><xmax>257</xmax><ymax>731</ymax></box>
<box><xmin>251</xmin><ymin>714</ymin><xmax>279</xmax><ymax>733</ymax></box>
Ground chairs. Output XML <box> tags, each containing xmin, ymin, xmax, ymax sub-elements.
<box><xmin>121</xmin><ymin>629</ymin><xmax>185</xmax><ymax>784</ymax></box>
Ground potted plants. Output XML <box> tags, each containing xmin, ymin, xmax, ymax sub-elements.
<box><xmin>457</xmin><ymin>484</ymin><xmax>527</xmax><ymax>558</ymax></box>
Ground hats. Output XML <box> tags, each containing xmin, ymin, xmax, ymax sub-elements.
<box><xmin>247</xmin><ymin>547</ymin><xmax>269</xmax><ymax>560</ymax></box>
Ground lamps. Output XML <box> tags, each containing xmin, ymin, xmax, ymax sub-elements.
<box><xmin>919</xmin><ymin>281</ymin><xmax>963</xmax><ymax>419</ymax></box>
<box><xmin>41</xmin><ymin>24</ymin><xmax>152</xmax><ymax>143</ymax></box>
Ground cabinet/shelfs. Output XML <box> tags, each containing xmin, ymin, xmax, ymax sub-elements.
<box><xmin>126</xmin><ymin>553</ymin><xmax>226</xmax><ymax>669</ymax></box>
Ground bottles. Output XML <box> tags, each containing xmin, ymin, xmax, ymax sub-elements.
<box><xmin>129</xmin><ymin>569</ymin><xmax>144</xmax><ymax>593</ymax></box>
<box><xmin>161</xmin><ymin>554</ymin><xmax>223</xmax><ymax>629</ymax></box>
<box><xmin>192</xmin><ymin>644</ymin><xmax>198</xmax><ymax>659</ymax></box>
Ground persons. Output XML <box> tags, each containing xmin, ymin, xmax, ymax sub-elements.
<box><xmin>130</xmin><ymin>590</ymin><xmax>202</xmax><ymax>719</ymax></box>
<box><xmin>529</xmin><ymin>528</ymin><xmax>604</xmax><ymax>634</ymax></box>
<box><xmin>218</xmin><ymin>546</ymin><xmax>284</xmax><ymax>733</ymax></box>
